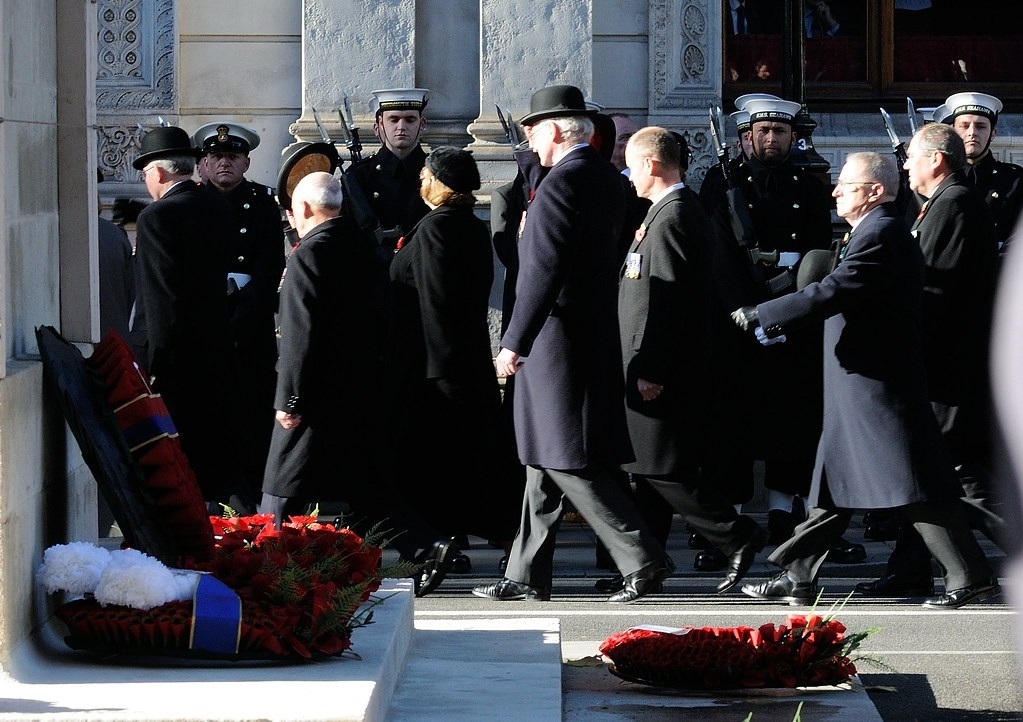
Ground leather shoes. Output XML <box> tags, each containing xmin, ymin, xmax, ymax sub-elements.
<box><xmin>687</xmin><ymin>530</ymin><xmax>714</xmax><ymax>550</ymax></box>
<box><xmin>717</xmin><ymin>528</ymin><xmax>772</xmax><ymax>595</ymax></box>
<box><xmin>863</xmin><ymin>522</ymin><xmax>899</xmax><ymax>540</ymax></box>
<box><xmin>414</xmin><ymin>539</ymin><xmax>453</xmax><ymax>597</ymax></box>
<box><xmin>921</xmin><ymin>575</ymin><xmax>1002</xmax><ymax>608</ymax></box>
<box><xmin>471</xmin><ymin>577</ymin><xmax>551</xmax><ymax>602</ymax></box>
<box><xmin>825</xmin><ymin>537</ymin><xmax>867</xmax><ymax>564</ymax></box>
<box><xmin>606</xmin><ymin>555</ymin><xmax>677</xmax><ymax>604</ymax></box>
<box><xmin>862</xmin><ymin>509</ymin><xmax>884</xmax><ymax>525</ymax></box>
<box><xmin>595</xmin><ymin>571</ymin><xmax>664</xmax><ymax>594</ymax></box>
<box><xmin>854</xmin><ymin>570</ymin><xmax>936</xmax><ymax>598</ymax></box>
<box><xmin>694</xmin><ymin>550</ymin><xmax>721</xmax><ymax>570</ymax></box>
<box><xmin>497</xmin><ymin>555</ymin><xmax>510</xmax><ymax>572</ymax></box>
<box><xmin>741</xmin><ymin>570</ymin><xmax>817</xmax><ymax>607</ymax></box>
<box><xmin>449</xmin><ymin>549</ymin><xmax>472</xmax><ymax>573</ymax></box>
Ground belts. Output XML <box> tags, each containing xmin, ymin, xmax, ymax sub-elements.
<box><xmin>227</xmin><ymin>273</ymin><xmax>251</xmax><ymax>289</ymax></box>
<box><xmin>777</xmin><ymin>252</ymin><xmax>801</xmax><ymax>269</ymax></box>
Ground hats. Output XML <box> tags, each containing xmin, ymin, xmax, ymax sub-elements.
<box><xmin>190</xmin><ymin>122</ymin><xmax>261</xmax><ymax>156</ymax></box>
<box><xmin>519</xmin><ymin>84</ymin><xmax>606</xmax><ymax>129</ymax></box>
<box><xmin>276</xmin><ymin>142</ymin><xmax>338</xmax><ymax>211</ymax></box>
<box><xmin>426</xmin><ymin>145</ymin><xmax>482</xmax><ymax>194</ymax></box>
<box><xmin>795</xmin><ymin>239</ymin><xmax>843</xmax><ymax>292</ymax></box>
<box><xmin>916</xmin><ymin>91</ymin><xmax>1004</xmax><ymax>126</ymax></box>
<box><xmin>132</xmin><ymin>126</ymin><xmax>203</xmax><ymax>171</ymax></box>
<box><xmin>368</xmin><ymin>88</ymin><xmax>430</xmax><ymax>116</ymax></box>
<box><xmin>730</xmin><ymin>93</ymin><xmax>801</xmax><ymax>132</ymax></box>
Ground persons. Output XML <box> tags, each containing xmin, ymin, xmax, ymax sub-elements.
<box><xmin>97</xmin><ymin>87</ymin><xmax>1023</xmax><ymax>611</ymax></box>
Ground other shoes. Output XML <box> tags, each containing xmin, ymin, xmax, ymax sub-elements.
<box><xmin>596</xmin><ymin>547</ymin><xmax>616</xmax><ymax>570</ymax></box>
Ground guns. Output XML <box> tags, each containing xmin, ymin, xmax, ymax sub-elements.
<box><xmin>708</xmin><ymin>100</ymin><xmax>794</xmax><ymax>294</ymax></box>
<box><xmin>879</xmin><ymin>96</ymin><xmax>926</xmax><ymax>229</ymax></box>
<box><xmin>310</xmin><ymin>90</ymin><xmax>403</xmax><ymax>254</ymax></box>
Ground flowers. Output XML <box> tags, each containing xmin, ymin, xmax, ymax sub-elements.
<box><xmin>527</xmin><ymin>190</ymin><xmax>534</xmax><ymax>203</ymax></box>
<box><xmin>89</xmin><ymin>328</ymin><xmax>218</xmax><ymax>566</ymax></box>
<box><xmin>291</xmin><ymin>241</ymin><xmax>299</xmax><ymax>252</ymax></box>
<box><xmin>396</xmin><ymin>236</ymin><xmax>405</xmax><ymax>250</ymax></box>
<box><xmin>600</xmin><ymin>587</ymin><xmax>908</xmax><ymax>698</ymax></box>
<box><xmin>32</xmin><ymin>506</ymin><xmax>435</xmax><ymax>662</ymax></box>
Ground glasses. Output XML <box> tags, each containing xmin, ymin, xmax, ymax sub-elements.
<box><xmin>834</xmin><ymin>179</ymin><xmax>876</xmax><ymax>191</ymax></box>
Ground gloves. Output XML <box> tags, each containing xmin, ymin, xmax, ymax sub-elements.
<box><xmin>754</xmin><ymin>324</ymin><xmax>787</xmax><ymax>346</ymax></box>
